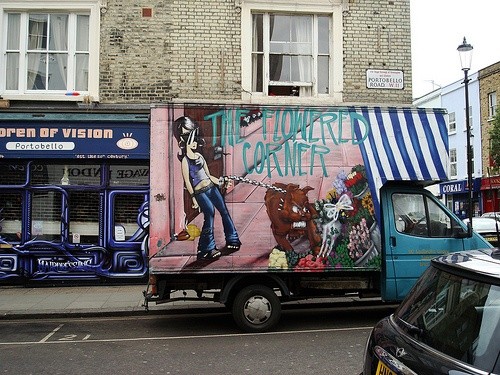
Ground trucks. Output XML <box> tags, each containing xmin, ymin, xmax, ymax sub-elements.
<box><xmin>140</xmin><ymin>101</ymin><xmax>495</xmax><ymax>333</ymax></box>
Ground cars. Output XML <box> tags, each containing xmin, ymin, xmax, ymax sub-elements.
<box><xmin>480</xmin><ymin>211</ymin><xmax>500</xmax><ymax>222</ymax></box>
<box><xmin>462</xmin><ymin>217</ymin><xmax>500</xmax><ymax>248</ymax></box>
<box><xmin>358</xmin><ymin>247</ymin><xmax>500</xmax><ymax>375</ymax></box>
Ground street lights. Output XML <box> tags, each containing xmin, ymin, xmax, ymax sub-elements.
<box><xmin>455</xmin><ymin>35</ymin><xmax>474</xmax><ymax>216</ymax></box>
